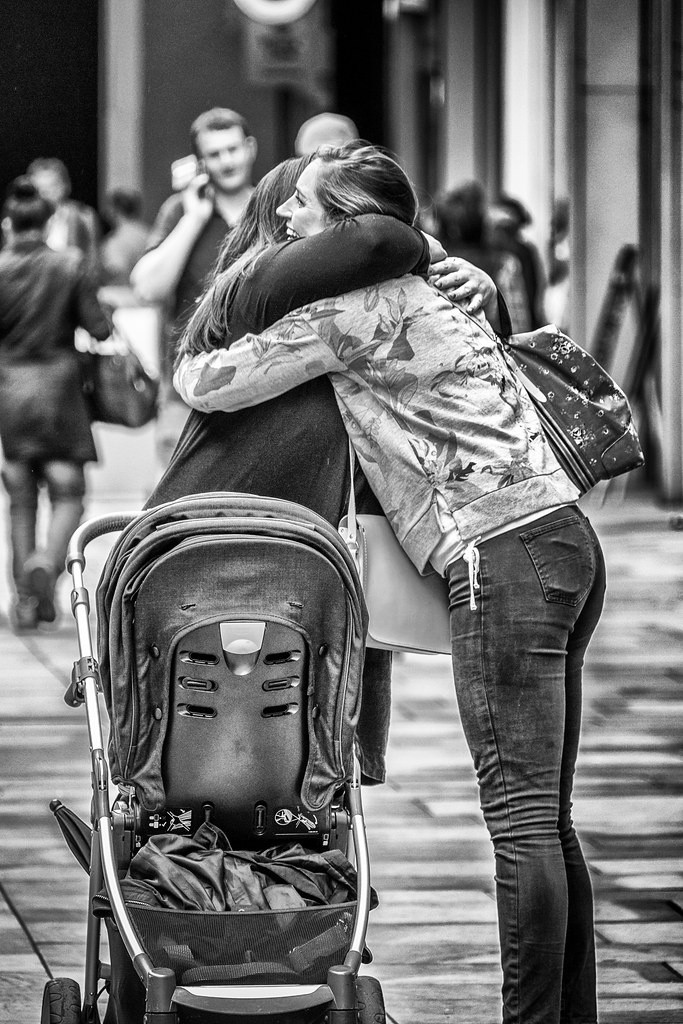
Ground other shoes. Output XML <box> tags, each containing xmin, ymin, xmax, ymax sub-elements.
<box><xmin>25</xmin><ymin>556</ymin><xmax>57</xmax><ymax>623</ymax></box>
<box><xmin>15</xmin><ymin>601</ymin><xmax>40</xmax><ymax>631</ymax></box>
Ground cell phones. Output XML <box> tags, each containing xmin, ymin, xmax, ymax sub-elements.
<box><xmin>196</xmin><ymin>159</ymin><xmax>207</xmax><ymax>198</ymax></box>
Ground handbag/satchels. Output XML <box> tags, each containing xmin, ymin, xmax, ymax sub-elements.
<box><xmin>337</xmin><ymin>512</ymin><xmax>453</xmax><ymax>656</ymax></box>
<box><xmin>87</xmin><ymin>316</ymin><xmax>160</xmax><ymax>428</ymax></box>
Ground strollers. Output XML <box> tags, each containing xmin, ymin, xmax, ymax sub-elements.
<box><xmin>43</xmin><ymin>491</ymin><xmax>393</xmax><ymax>1024</ymax></box>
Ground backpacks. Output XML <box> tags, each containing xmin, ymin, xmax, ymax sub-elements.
<box><xmin>420</xmin><ymin>281</ymin><xmax>646</xmax><ymax>502</ymax></box>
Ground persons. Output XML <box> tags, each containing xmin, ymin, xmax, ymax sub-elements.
<box><xmin>1</xmin><ymin>183</ymin><xmax>114</xmax><ymax>630</ymax></box>
<box><xmin>146</xmin><ymin>156</ymin><xmax>511</xmax><ymax>785</ymax></box>
<box><xmin>418</xmin><ymin>179</ymin><xmax>552</xmax><ymax>344</ymax></box>
<box><xmin>173</xmin><ymin>139</ymin><xmax>611</xmax><ymax>1023</ymax></box>
<box><xmin>18</xmin><ymin>159</ymin><xmax>151</xmax><ymax>432</ymax></box>
<box><xmin>130</xmin><ymin>106</ymin><xmax>261</xmax><ymax>472</ymax></box>
